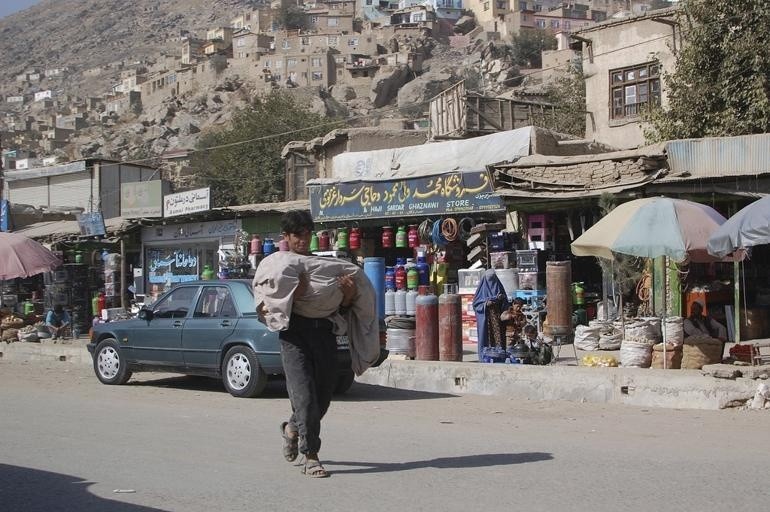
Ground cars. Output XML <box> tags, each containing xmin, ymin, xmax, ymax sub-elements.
<box><xmin>86</xmin><ymin>278</ymin><xmax>389</xmax><ymax>398</ymax></box>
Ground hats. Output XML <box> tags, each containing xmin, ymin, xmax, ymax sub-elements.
<box><xmin>693</xmin><ymin>299</ymin><xmax>704</xmax><ymax>308</ymax></box>
<box><xmin>54</xmin><ymin>304</ymin><xmax>64</xmax><ymax>314</ymax></box>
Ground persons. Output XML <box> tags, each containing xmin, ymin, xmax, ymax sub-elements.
<box><xmin>472</xmin><ymin>268</ymin><xmax>552</xmax><ymax>366</ymax></box>
<box><xmin>252</xmin><ymin>209</ymin><xmax>381</xmax><ymax>479</ymax></box>
<box><xmin>45</xmin><ymin>304</ymin><xmax>71</xmax><ymax>340</ymax></box>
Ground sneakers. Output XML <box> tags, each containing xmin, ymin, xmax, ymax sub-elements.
<box><xmin>52</xmin><ymin>335</ymin><xmax>64</xmax><ymax>340</ymax></box>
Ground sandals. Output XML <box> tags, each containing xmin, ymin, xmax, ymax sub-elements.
<box><xmin>305</xmin><ymin>461</ymin><xmax>328</xmax><ymax>478</ymax></box>
<box><xmin>280</xmin><ymin>421</ymin><xmax>298</xmax><ymax>462</ymax></box>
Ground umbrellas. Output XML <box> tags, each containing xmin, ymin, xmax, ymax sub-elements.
<box><xmin>570</xmin><ymin>195</ymin><xmax>770</xmax><ymax>369</ymax></box>
<box><xmin>0</xmin><ymin>230</ymin><xmax>63</xmax><ymax>281</ymax></box>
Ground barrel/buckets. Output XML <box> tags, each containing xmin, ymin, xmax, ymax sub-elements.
<box><xmin>251</xmin><ymin>221</ymin><xmax>419</xmax><ymax>256</ymax></box>
